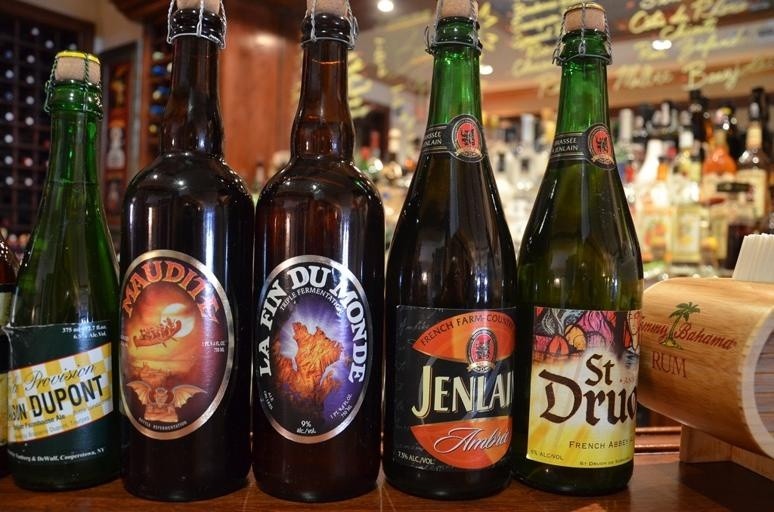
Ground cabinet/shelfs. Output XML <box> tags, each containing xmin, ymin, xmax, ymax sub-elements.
<box><xmin>0</xmin><ymin>0</ymin><xmax>175</xmax><ymax>233</ymax></box>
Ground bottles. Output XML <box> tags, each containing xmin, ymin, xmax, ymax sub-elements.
<box><xmin>0</xmin><ymin>52</ymin><xmax>124</xmax><ymax>491</ymax></box>
<box><xmin>126</xmin><ymin>1</ymin><xmax>255</xmax><ymax>502</ymax></box>
<box><xmin>255</xmin><ymin>1</ymin><xmax>386</xmax><ymax>507</ymax></box>
<box><xmin>382</xmin><ymin>1</ymin><xmax>520</xmax><ymax>504</ymax></box>
<box><xmin>511</xmin><ymin>0</ymin><xmax>646</xmax><ymax>496</ymax></box>
<box><xmin>362</xmin><ymin>85</ymin><xmax>773</xmax><ymax>276</ymax></box>
